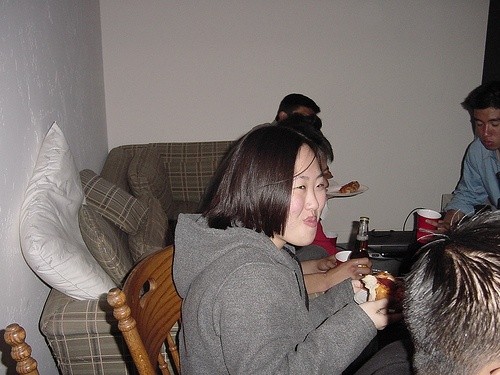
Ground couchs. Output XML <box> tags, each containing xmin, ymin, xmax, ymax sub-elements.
<box><xmin>39</xmin><ymin>141</ymin><xmax>235</xmax><ymax>375</ymax></box>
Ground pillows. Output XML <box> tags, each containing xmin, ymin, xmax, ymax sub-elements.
<box><xmin>20</xmin><ymin>120</ymin><xmax>172</xmax><ymax>307</ymax></box>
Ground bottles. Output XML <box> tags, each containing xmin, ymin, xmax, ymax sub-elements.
<box><xmin>351</xmin><ymin>249</ymin><xmax>369</xmax><ymax>277</ymax></box>
<box><xmin>354</xmin><ymin>216</ymin><xmax>369</xmax><ymax>248</ymax></box>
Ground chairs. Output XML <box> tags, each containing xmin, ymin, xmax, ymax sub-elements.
<box><xmin>106</xmin><ymin>244</ymin><xmax>183</xmax><ymax>375</ymax></box>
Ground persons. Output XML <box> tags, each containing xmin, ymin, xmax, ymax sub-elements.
<box><xmin>351</xmin><ymin>209</ymin><xmax>500</xmax><ymax>375</ymax></box>
<box><xmin>419</xmin><ymin>82</ymin><xmax>500</xmax><ymax>241</ymax></box>
<box><xmin>172</xmin><ymin>93</ymin><xmax>389</xmax><ymax>375</ymax></box>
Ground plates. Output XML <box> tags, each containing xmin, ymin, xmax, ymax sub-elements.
<box><xmin>337</xmin><ymin>250</ymin><xmax>352</xmax><ymax>262</ymax></box>
<box><xmin>326</xmin><ymin>185</ymin><xmax>368</xmax><ymax>197</ymax></box>
<box><xmin>354</xmin><ymin>277</ymin><xmax>407</xmax><ymax>313</ymax></box>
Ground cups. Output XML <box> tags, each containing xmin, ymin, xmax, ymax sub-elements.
<box><xmin>415</xmin><ymin>208</ymin><xmax>441</xmax><ymax>244</ymax></box>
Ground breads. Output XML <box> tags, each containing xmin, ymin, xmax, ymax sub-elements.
<box><xmin>339</xmin><ymin>180</ymin><xmax>360</xmax><ymax>193</ymax></box>
<box><xmin>357</xmin><ymin>265</ymin><xmax>404</xmax><ymax>301</ymax></box>
